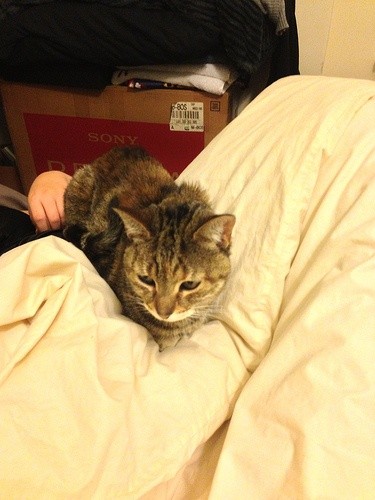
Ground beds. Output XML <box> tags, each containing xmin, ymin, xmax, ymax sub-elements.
<box><xmin>0</xmin><ymin>0</ymin><xmax>375</xmax><ymax>500</ymax></box>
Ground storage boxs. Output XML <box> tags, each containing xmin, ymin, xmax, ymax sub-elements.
<box><xmin>0</xmin><ymin>78</ymin><xmax>232</xmax><ymax>196</ymax></box>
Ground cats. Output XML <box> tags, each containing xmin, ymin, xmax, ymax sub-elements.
<box><xmin>60</xmin><ymin>143</ymin><xmax>237</xmax><ymax>353</ymax></box>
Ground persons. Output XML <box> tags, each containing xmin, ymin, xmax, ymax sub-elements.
<box><xmin>0</xmin><ymin>170</ymin><xmax>74</xmax><ymax>256</ymax></box>
